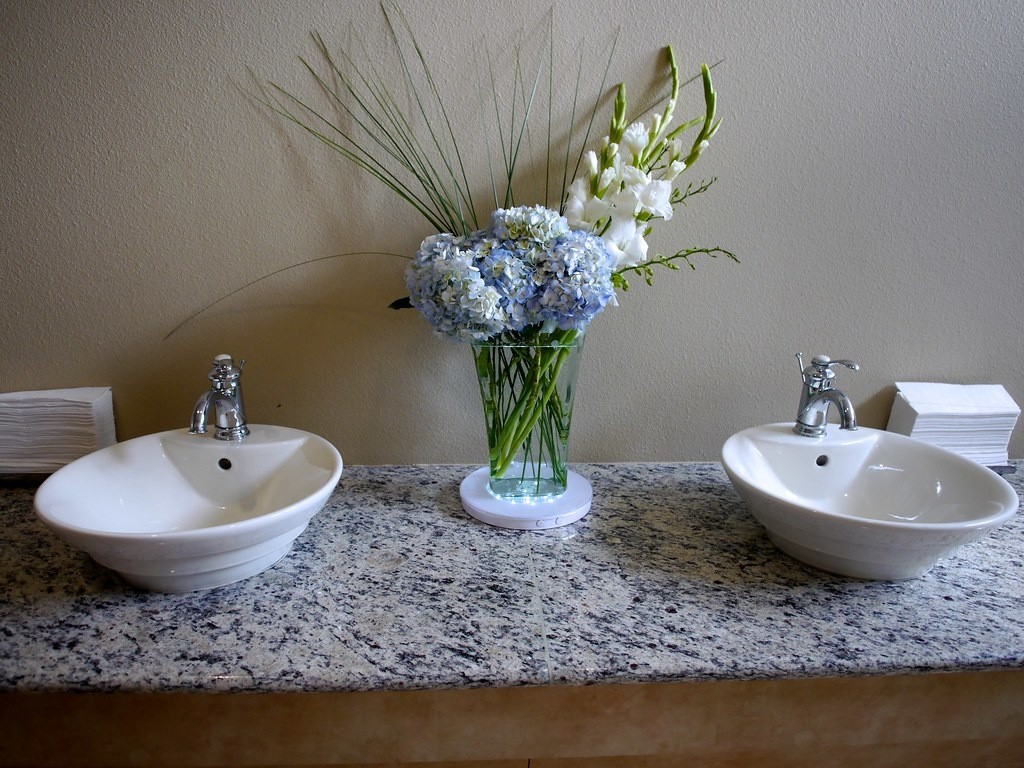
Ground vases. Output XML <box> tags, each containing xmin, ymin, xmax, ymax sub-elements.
<box><xmin>470</xmin><ymin>329</ymin><xmax>586</xmax><ymax>496</ymax></box>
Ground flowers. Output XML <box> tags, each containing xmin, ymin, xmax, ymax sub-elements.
<box><xmin>161</xmin><ymin>0</ymin><xmax>741</xmax><ymax>346</ymax></box>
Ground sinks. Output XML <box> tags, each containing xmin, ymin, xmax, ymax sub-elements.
<box><xmin>719</xmin><ymin>417</ymin><xmax>1021</xmax><ymax>580</ymax></box>
<box><xmin>32</xmin><ymin>420</ymin><xmax>344</xmax><ymax>596</ymax></box>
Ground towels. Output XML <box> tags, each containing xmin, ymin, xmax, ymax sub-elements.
<box><xmin>0</xmin><ymin>383</ymin><xmax>116</xmax><ymax>475</ymax></box>
<box><xmin>883</xmin><ymin>381</ymin><xmax>1022</xmax><ymax>468</ymax></box>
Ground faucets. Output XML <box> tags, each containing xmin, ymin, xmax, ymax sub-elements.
<box><xmin>187</xmin><ymin>351</ymin><xmax>251</xmax><ymax>441</ymax></box>
<box><xmin>793</xmin><ymin>348</ymin><xmax>861</xmax><ymax>438</ymax></box>
